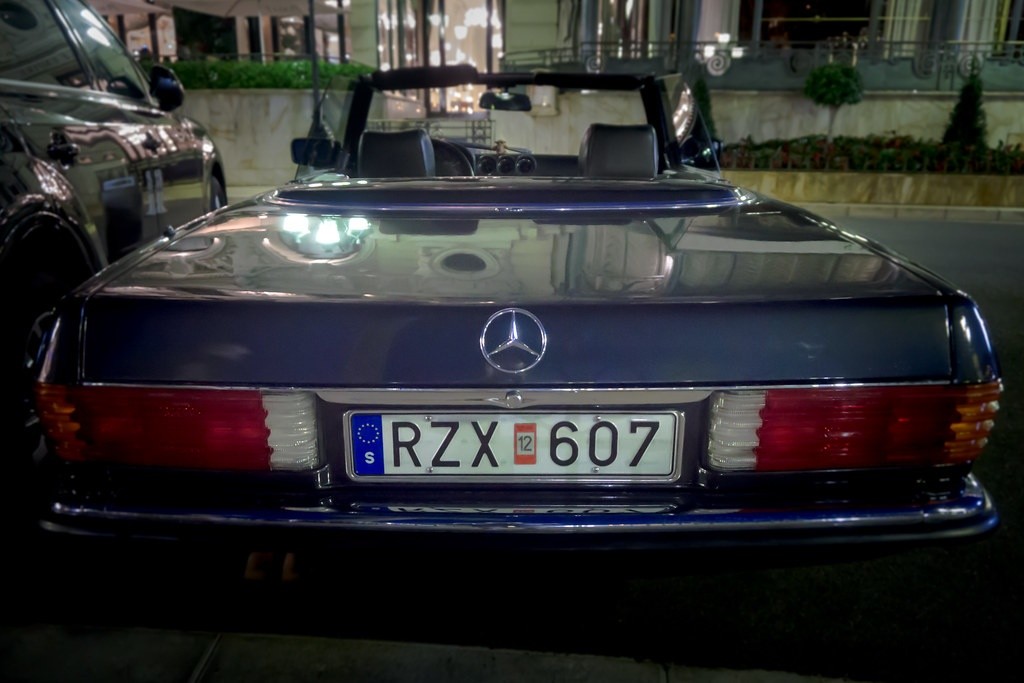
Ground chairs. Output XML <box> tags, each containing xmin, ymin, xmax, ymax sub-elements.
<box><xmin>578</xmin><ymin>122</ymin><xmax>658</xmax><ymax>179</ymax></box>
<box><xmin>357</xmin><ymin>128</ymin><xmax>436</xmax><ymax>179</ymax></box>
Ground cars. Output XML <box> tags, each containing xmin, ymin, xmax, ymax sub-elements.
<box><xmin>1</xmin><ymin>0</ymin><xmax>229</xmax><ymax>507</ymax></box>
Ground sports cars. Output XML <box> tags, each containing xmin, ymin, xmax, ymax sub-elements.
<box><xmin>22</xmin><ymin>61</ymin><xmax>1003</xmax><ymax>545</ymax></box>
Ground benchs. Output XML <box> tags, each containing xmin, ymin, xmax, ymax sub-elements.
<box><xmin>278</xmin><ymin>177</ymin><xmax>735</xmax><ymax>205</ymax></box>
